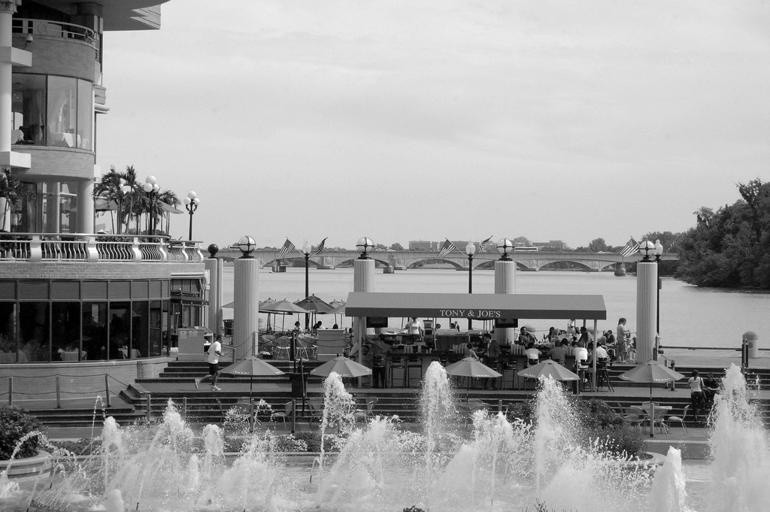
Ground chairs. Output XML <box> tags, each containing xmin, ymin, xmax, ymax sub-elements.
<box><xmin>459</xmin><ymin>397</ymin><xmax>533</xmax><ymax>428</ymax></box>
<box><xmin>600</xmin><ymin>400</ymin><xmax>690</xmax><ymax>438</ymax></box>
<box><xmin>387</xmin><ymin>337</ymin><xmax>618</xmax><ymax>393</ymax></box>
<box><xmin>304</xmin><ymin>394</ymin><xmax>379</xmax><ymax>433</ymax></box>
<box><xmin>214</xmin><ymin>396</ymin><xmax>293</xmax><ymax>431</ymax></box>
<box><xmin>259</xmin><ymin>330</ymin><xmax>318</xmax><ymax>360</ymax></box>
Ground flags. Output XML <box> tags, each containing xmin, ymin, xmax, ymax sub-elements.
<box><xmin>278</xmin><ymin>238</ymin><xmax>295</xmax><ymax>259</ymax></box>
<box><xmin>618</xmin><ymin>237</ymin><xmax>642</xmax><ymax>258</ymax></box>
<box><xmin>437</xmin><ymin>238</ymin><xmax>457</xmax><ymax>259</ymax></box>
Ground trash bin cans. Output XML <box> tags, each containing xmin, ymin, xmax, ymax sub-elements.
<box><xmin>372</xmin><ymin>354</ymin><xmax>388</xmax><ymax>388</ymax></box>
<box><xmin>290</xmin><ymin>373</ymin><xmax>308</xmax><ymax>398</ymax></box>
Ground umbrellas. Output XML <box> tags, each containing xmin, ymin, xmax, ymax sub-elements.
<box><xmin>220</xmin><ymin>292</ymin><xmax>346</xmax><ymax>335</ymax></box>
<box><xmin>218</xmin><ymin>353</ymin><xmax>286</xmax><ymax>405</ymax></box>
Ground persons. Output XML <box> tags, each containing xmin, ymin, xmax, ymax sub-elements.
<box><xmin>292</xmin><ymin>315</ymin><xmax>631</xmax><ymax>389</ymax></box>
<box><xmin>193</xmin><ymin>334</ymin><xmax>225</xmax><ymax>393</ymax></box>
<box><xmin>689</xmin><ymin>370</ymin><xmax>721</xmax><ymax>421</ymax></box>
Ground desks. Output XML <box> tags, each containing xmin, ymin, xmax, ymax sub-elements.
<box><xmin>54</xmin><ymin>132</ymin><xmax>81</xmax><ymax>148</ymax></box>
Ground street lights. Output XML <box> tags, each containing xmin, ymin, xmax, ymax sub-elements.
<box><xmin>303</xmin><ymin>239</ymin><xmax>314</xmax><ymax>330</ymax></box>
<box><xmin>464</xmin><ymin>239</ymin><xmax>477</xmax><ymax>331</ymax></box>
<box><xmin>143</xmin><ymin>174</ymin><xmax>159</xmax><ymax>240</ymax></box>
<box><xmin>183</xmin><ymin>189</ymin><xmax>201</xmax><ymax>242</ymax></box>
<box><xmin>653</xmin><ymin>238</ymin><xmax>665</xmax><ymax>333</ymax></box>
